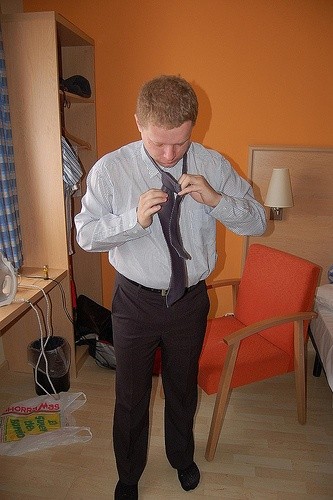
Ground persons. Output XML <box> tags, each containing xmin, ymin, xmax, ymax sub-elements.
<box><xmin>71</xmin><ymin>75</ymin><xmax>267</xmax><ymax>500</ymax></box>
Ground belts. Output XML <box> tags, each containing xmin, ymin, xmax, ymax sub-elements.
<box><xmin>128</xmin><ymin>276</ymin><xmax>200</xmax><ymax>296</ymax></box>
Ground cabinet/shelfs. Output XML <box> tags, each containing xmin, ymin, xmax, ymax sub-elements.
<box><xmin>0</xmin><ymin>11</ymin><xmax>102</xmax><ymax>380</ymax></box>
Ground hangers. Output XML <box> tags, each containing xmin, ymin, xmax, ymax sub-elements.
<box><xmin>62</xmin><ymin>129</ymin><xmax>91</xmax><ymax>150</ymax></box>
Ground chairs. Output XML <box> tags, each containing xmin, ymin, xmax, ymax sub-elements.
<box><xmin>159</xmin><ymin>244</ymin><xmax>322</xmax><ymax>463</ymax></box>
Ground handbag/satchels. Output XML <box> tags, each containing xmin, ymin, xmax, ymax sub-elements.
<box><xmin>75</xmin><ymin>295</ymin><xmax>116</xmax><ymax>371</ymax></box>
<box><xmin>59</xmin><ymin>75</ymin><xmax>92</xmax><ymax>99</ymax></box>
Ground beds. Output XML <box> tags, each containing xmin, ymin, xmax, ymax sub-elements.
<box><xmin>308</xmin><ymin>283</ymin><xmax>333</xmax><ymax>393</ymax></box>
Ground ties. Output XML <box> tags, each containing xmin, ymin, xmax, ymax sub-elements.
<box><xmin>143</xmin><ymin>145</ymin><xmax>192</xmax><ymax>310</ymax></box>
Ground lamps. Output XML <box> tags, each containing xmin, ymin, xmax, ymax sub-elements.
<box><xmin>264</xmin><ymin>168</ymin><xmax>293</xmax><ymax>220</ymax></box>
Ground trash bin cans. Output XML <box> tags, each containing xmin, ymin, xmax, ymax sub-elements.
<box><xmin>26</xmin><ymin>336</ymin><xmax>72</xmax><ymax>396</ymax></box>
